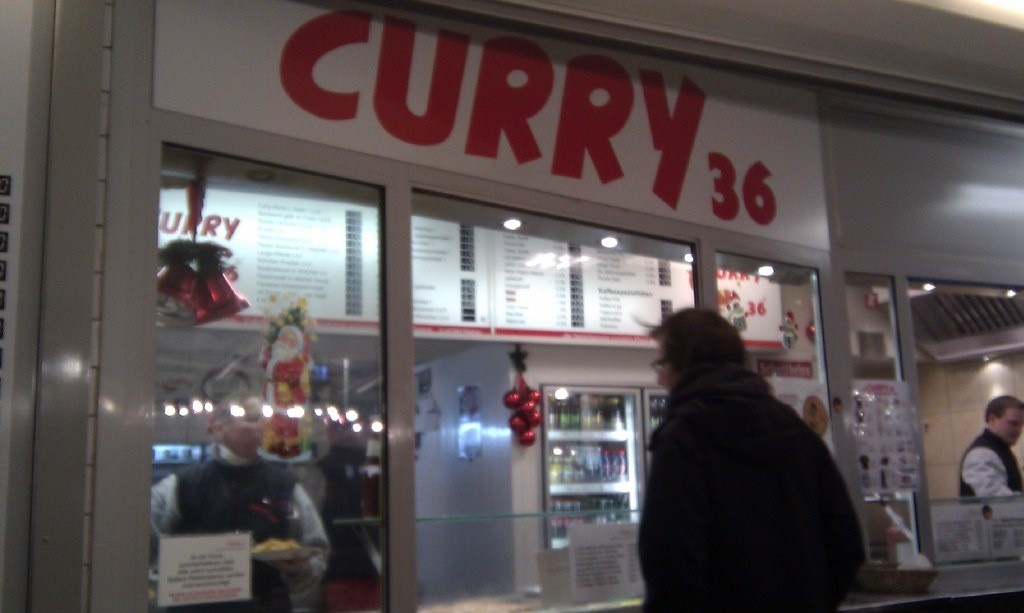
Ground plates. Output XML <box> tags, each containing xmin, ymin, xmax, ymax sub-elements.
<box><xmin>250</xmin><ymin>548</ymin><xmax>321</xmax><ymax>562</ymax></box>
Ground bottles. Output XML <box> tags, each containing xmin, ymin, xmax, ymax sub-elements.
<box><xmin>650</xmin><ymin>396</ymin><xmax>666</xmax><ymax>426</ymax></box>
<box><xmin>549</xmin><ymin>394</ymin><xmax>625</xmax><ymax>431</ymax></box>
<box><xmin>549</xmin><ymin>497</ymin><xmax>629</xmax><ymax>538</ymax></box>
<box><xmin>548</xmin><ymin>446</ymin><xmax>626</xmax><ymax>485</ymax></box>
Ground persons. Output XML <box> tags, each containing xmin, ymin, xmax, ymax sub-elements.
<box><xmin>143</xmin><ymin>382</ymin><xmax>331</xmax><ymax>612</ymax></box>
<box><xmin>635</xmin><ymin>307</ymin><xmax>865</xmax><ymax>612</ymax></box>
<box><xmin>958</xmin><ymin>396</ymin><xmax>1024</xmax><ymax>500</ymax></box>
